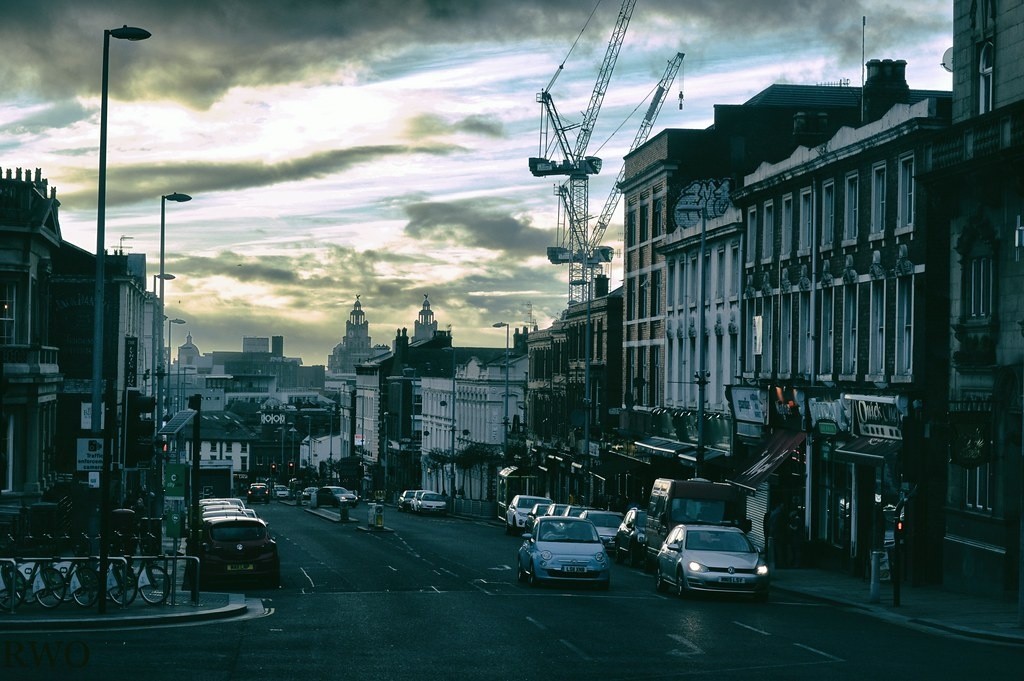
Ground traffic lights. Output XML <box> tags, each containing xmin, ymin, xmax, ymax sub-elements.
<box><xmin>289</xmin><ymin>461</ymin><xmax>294</xmax><ymax>473</ymax></box>
<box><xmin>271</xmin><ymin>464</ymin><xmax>276</xmax><ymax>473</ymax></box>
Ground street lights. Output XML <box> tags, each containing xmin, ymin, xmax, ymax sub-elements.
<box><xmin>152</xmin><ymin>273</ymin><xmax>176</xmax><ymax>481</ymax></box>
<box><xmin>156</xmin><ymin>192</ymin><xmax>192</xmax><ymax>520</ymax></box>
<box><xmin>493</xmin><ymin>321</ymin><xmax>508</xmax><ymax>467</ymax></box>
<box><xmin>402</xmin><ymin>367</ymin><xmax>418</xmax><ymax>489</ymax></box>
<box><xmin>441</xmin><ymin>345</ymin><xmax>456</xmax><ymax>497</ymax></box>
<box><xmin>166</xmin><ymin>318</ymin><xmax>188</xmax><ymax>479</ymax></box>
<box><xmin>94</xmin><ymin>25</ymin><xmax>152</xmax><ymax>615</ymax></box>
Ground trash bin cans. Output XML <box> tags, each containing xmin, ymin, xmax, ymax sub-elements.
<box><xmin>109</xmin><ymin>508</ymin><xmax>136</xmax><ymax>556</ymax></box>
<box><xmin>367</xmin><ymin>503</ymin><xmax>384</xmax><ymax>531</ymax></box>
<box><xmin>296</xmin><ymin>491</ymin><xmax>302</xmax><ymax>505</ymax></box>
<box><xmin>140</xmin><ymin>518</ymin><xmax>163</xmax><ymax>557</ymax></box>
<box><xmin>340</xmin><ymin>500</ymin><xmax>349</xmax><ymax>520</ymax></box>
<box><xmin>273</xmin><ymin>489</ymin><xmax>277</xmax><ymax>499</ymax></box>
<box><xmin>310</xmin><ymin>493</ymin><xmax>317</xmax><ymax>508</ymax></box>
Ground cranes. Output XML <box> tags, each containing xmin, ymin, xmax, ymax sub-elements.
<box><xmin>528</xmin><ymin>0</ymin><xmax>685</xmax><ymax>311</ymax></box>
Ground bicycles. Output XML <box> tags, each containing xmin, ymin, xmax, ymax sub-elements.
<box><xmin>0</xmin><ymin>530</ymin><xmax>171</xmax><ymax>611</ymax></box>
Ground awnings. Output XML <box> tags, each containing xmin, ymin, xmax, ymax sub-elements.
<box><xmin>590</xmin><ymin>465</ymin><xmax>621</xmax><ymax>480</ymax></box>
<box><xmin>678</xmin><ymin>448</ymin><xmax>726</xmax><ymax>467</ymax></box>
<box><xmin>634</xmin><ymin>437</ymin><xmax>694</xmax><ymax>456</ymax></box>
<box><xmin>726</xmin><ymin>432</ymin><xmax>807</xmax><ymax>492</ymax></box>
<box><xmin>538</xmin><ymin>457</ymin><xmax>559</xmax><ymax>471</ymax></box>
<box><xmin>836</xmin><ymin>436</ymin><xmax>903</xmax><ymax>458</ymax></box>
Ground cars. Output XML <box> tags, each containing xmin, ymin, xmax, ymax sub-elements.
<box><xmin>194</xmin><ymin>497</ymin><xmax>281</xmax><ymax>589</ymax></box>
<box><xmin>517</xmin><ymin>516</ymin><xmax>610</xmax><ymax>586</ymax></box>
<box><xmin>657</xmin><ymin>525</ymin><xmax>769</xmax><ymax>600</ymax></box>
<box><xmin>303</xmin><ymin>485</ymin><xmax>358</xmax><ymax>508</ymax></box>
<box><xmin>399</xmin><ymin>489</ymin><xmax>448</xmax><ymax>514</ymax></box>
<box><xmin>506</xmin><ymin>494</ymin><xmax>621</xmax><ymax>553</ymax></box>
<box><xmin>248</xmin><ymin>483</ymin><xmax>289</xmax><ymax>503</ymax></box>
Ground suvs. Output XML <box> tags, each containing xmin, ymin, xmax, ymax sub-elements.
<box><xmin>613</xmin><ymin>506</ymin><xmax>651</xmax><ymax>568</ymax></box>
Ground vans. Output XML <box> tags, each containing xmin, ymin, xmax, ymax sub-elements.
<box><xmin>644</xmin><ymin>477</ymin><xmax>751</xmax><ymax>569</ymax></box>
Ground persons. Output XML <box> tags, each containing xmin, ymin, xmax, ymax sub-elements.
<box><xmin>442</xmin><ymin>490</ymin><xmax>448</xmax><ymax>497</ymax></box>
<box><xmin>121</xmin><ymin>486</ymin><xmax>151</xmax><ymax>556</ymax></box>
<box><xmin>458</xmin><ymin>486</ymin><xmax>465</xmax><ymax>499</ymax></box>
<box><xmin>769</xmin><ymin>505</ymin><xmax>802</xmax><ymax>568</ymax></box>
